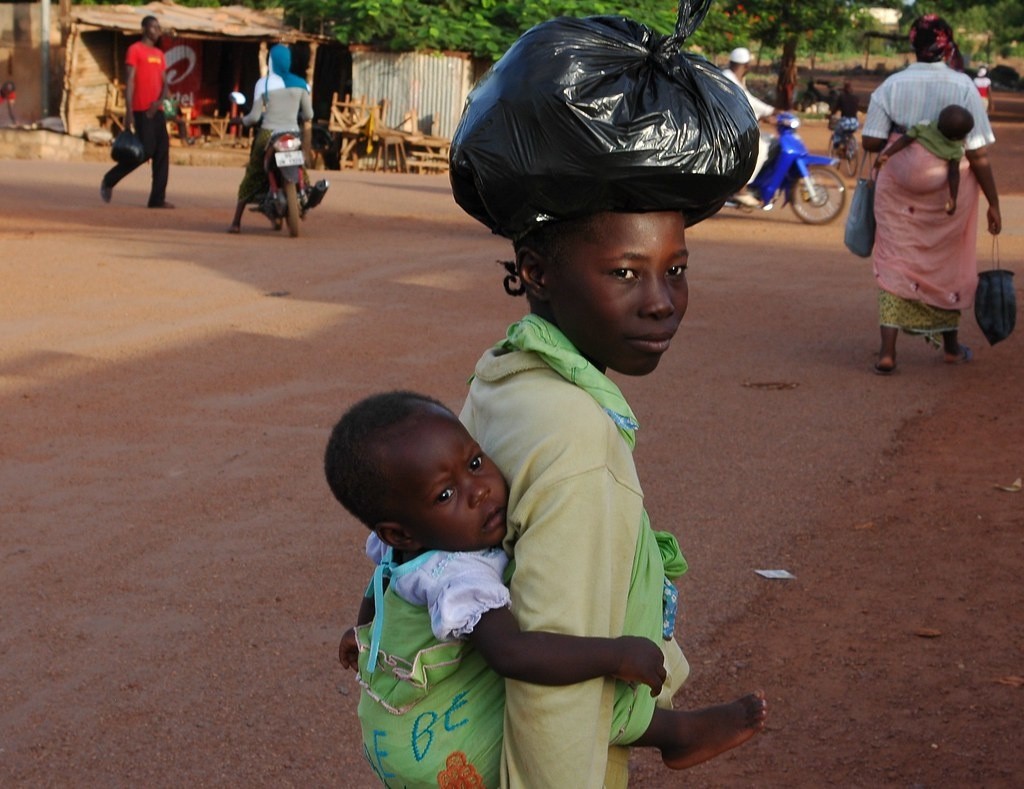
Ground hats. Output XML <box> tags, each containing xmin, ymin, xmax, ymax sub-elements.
<box><xmin>729</xmin><ymin>47</ymin><xmax>750</xmax><ymax>63</ymax></box>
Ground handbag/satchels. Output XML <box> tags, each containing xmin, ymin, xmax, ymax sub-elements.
<box><xmin>974</xmin><ymin>234</ymin><xmax>1015</xmax><ymax>346</ymax></box>
<box><xmin>111</xmin><ymin>129</ymin><xmax>145</xmax><ymax>164</ymax></box>
<box><xmin>450</xmin><ymin>0</ymin><xmax>760</xmax><ymax>244</ymax></box>
<box><xmin>844</xmin><ymin>151</ymin><xmax>880</xmax><ymax>257</ymax></box>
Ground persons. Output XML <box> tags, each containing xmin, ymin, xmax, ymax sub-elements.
<box><xmin>974</xmin><ymin>66</ymin><xmax>994</xmax><ymax>118</ymax></box>
<box><xmin>831</xmin><ymin>82</ymin><xmax>859</xmax><ymax>167</ymax></box>
<box><xmin>325</xmin><ymin>204</ymin><xmax>767</xmax><ymax>789</ymax></box>
<box><xmin>226</xmin><ymin>44</ymin><xmax>314</xmax><ymax>233</ymax></box>
<box><xmin>718</xmin><ymin>47</ymin><xmax>785</xmax><ymax>207</ymax></box>
<box><xmin>863</xmin><ymin>13</ymin><xmax>1002</xmax><ymax>374</ymax></box>
<box><xmin>102</xmin><ymin>16</ymin><xmax>173</xmax><ymax>208</ymax></box>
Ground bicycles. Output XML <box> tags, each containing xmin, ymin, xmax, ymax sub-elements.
<box><xmin>826</xmin><ymin>114</ymin><xmax>859</xmax><ymax>177</ymax></box>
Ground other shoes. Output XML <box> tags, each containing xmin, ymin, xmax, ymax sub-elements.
<box><xmin>101</xmin><ymin>179</ymin><xmax>112</xmax><ymax>203</ymax></box>
<box><xmin>733</xmin><ymin>195</ymin><xmax>760</xmax><ymax>206</ymax></box>
<box><xmin>148</xmin><ymin>202</ymin><xmax>176</xmax><ymax>209</ymax></box>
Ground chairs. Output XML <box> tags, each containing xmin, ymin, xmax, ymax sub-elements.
<box><xmin>180</xmin><ymin>105</ymin><xmax>232</xmax><ymax>142</ymax></box>
<box><xmin>103</xmin><ymin>78</ymin><xmax>127</xmax><ymax>130</ymax></box>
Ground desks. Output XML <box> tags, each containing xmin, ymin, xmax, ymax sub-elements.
<box><xmin>327</xmin><ymin>93</ymin><xmax>451</xmax><ymax>175</ymax></box>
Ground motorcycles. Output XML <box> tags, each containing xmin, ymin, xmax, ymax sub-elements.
<box><xmin>229</xmin><ymin>90</ymin><xmax>334</xmax><ymax>236</ymax></box>
<box><xmin>722</xmin><ymin>110</ymin><xmax>846</xmax><ymax>227</ymax></box>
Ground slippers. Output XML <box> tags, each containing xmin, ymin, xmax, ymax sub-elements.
<box><xmin>873</xmin><ymin>362</ymin><xmax>896</xmax><ymax>375</ymax></box>
<box><xmin>942</xmin><ymin>344</ymin><xmax>973</xmax><ymax>365</ymax></box>
